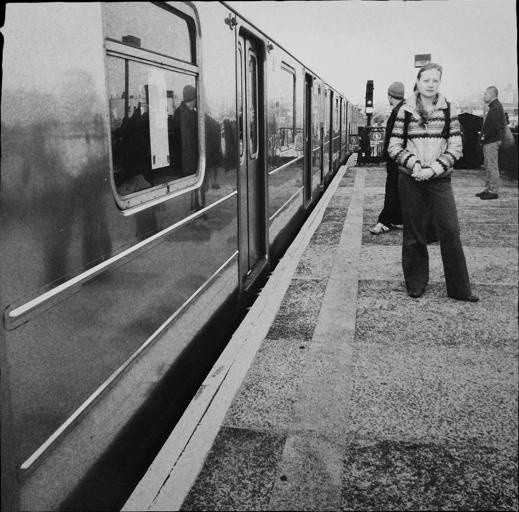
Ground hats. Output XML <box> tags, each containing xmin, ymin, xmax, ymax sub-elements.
<box><xmin>388</xmin><ymin>82</ymin><xmax>404</xmax><ymax>99</ymax></box>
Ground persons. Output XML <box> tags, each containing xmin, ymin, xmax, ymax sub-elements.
<box><xmin>474</xmin><ymin>85</ymin><xmax>506</xmax><ymax>200</ymax></box>
<box><xmin>369</xmin><ymin>81</ymin><xmax>407</xmax><ymax>235</ymax></box>
<box><xmin>386</xmin><ymin>62</ymin><xmax>480</xmax><ymax>303</ymax></box>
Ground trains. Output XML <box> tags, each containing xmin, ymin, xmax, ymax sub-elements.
<box><xmin>0</xmin><ymin>0</ymin><xmax>365</xmax><ymax>511</ymax></box>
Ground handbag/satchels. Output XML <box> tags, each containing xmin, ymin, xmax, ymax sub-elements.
<box><xmin>501</xmin><ymin>123</ymin><xmax>515</xmax><ymax>150</ymax></box>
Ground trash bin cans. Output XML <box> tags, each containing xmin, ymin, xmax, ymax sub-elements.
<box><xmin>358</xmin><ymin>126</ymin><xmax>385</xmax><ymax>166</ymax></box>
<box><xmin>453</xmin><ymin>113</ymin><xmax>482</xmax><ymax>169</ymax></box>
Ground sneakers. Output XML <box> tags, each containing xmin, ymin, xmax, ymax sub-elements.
<box><xmin>371</xmin><ymin>222</ymin><xmax>403</xmax><ymax>234</ymax></box>
<box><xmin>476</xmin><ymin>191</ymin><xmax>498</xmax><ymax>200</ymax></box>
<box><xmin>408</xmin><ymin>288</ymin><xmax>424</xmax><ymax>298</ymax></box>
<box><xmin>460</xmin><ymin>295</ymin><xmax>479</xmax><ymax>302</ymax></box>
